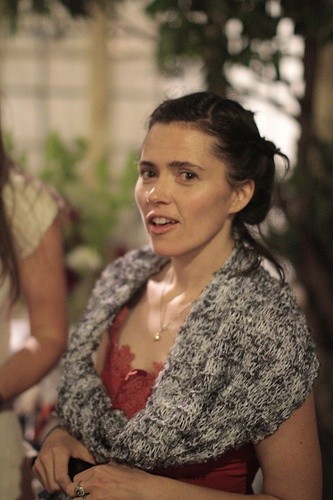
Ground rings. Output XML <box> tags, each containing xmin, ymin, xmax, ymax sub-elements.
<box><xmin>77</xmin><ymin>481</ymin><xmax>87</xmax><ymax>496</ymax></box>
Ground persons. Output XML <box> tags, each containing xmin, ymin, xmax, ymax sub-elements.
<box><xmin>20</xmin><ymin>92</ymin><xmax>324</xmax><ymax>500</ymax></box>
<box><xmin>0</xmin><ymin>120</ymin><xmax>76</xmax><ymax>500</ymax></box>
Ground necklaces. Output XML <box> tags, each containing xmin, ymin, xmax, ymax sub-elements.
<box><xmin>152</xmin><ymin>238</ymin><xmax>237</xmax><ymax>342</ymax></box>
